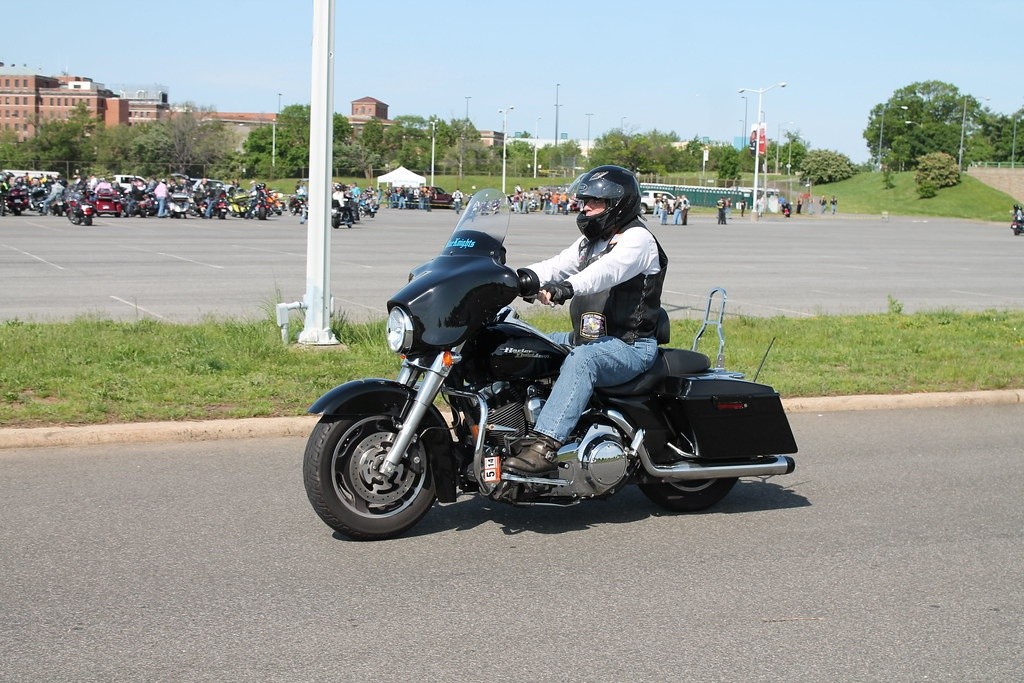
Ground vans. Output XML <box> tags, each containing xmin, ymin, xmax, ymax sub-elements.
<box><xmin>3</xmin><ymin>170</ymin><xmax>62</xmax><ymax>181</ymax></box>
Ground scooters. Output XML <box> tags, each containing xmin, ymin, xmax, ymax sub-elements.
<box><xmin>464</xmin><ymin>186</ymin><xmax>501</xmax><ymax>216</ymax></box>
<box><xmin>1010</xmin><ymin>216</ymin><xmax>1024</xmax><ymax>235</ymax></box>
<box><xmin>508</xmin><ymin>195</ymin><xmax>577</xmax><ymax>212</ymax></box>
<box><xmin>780</xmin><ymin>202</ymin><xmax>791</xmax><ymax>219</ymax></box>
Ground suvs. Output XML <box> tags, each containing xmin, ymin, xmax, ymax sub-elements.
<box><xmin>641</xmin><ymin>189</ymin><xmax>677</xmax><ymax>213</ymax></box>
<box><xmin>413</xmin><ymin>186</ymin><xmax>454</xmax><ymax>210</ymax></box>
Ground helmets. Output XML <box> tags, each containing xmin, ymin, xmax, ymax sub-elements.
<box><xmin>576</xmin><ymin>165</ymin><xmax>641</xmax><ymax>242</ymax></box>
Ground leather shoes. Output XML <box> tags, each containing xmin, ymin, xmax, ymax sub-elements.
<box><xmin>502</xmin><ymin>432</ymin><xmax>559</xmax><ymax>479</ymax></box>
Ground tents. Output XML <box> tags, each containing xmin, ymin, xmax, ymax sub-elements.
<box><xmin>377</xmin><ymin>166</ymin><xmax>425</xmax><ymax>189</ymax></box>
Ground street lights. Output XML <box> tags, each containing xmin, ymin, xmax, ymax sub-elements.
<box><xmin>585</xmin><ymin>113</ymin><xmax>595</xmax><ymax>152</ymax></box>
<box><xmin>465</xmin><ymin>96</ymin><xmax>472</xmax><ymax>134</ymax></box>
<box><xmin>273</xmin><ymin>119</ymin><xmax>276</xmax><ymax>166</ymax></box>
<box><xmin>621</xmin><ymin>116</ymin><xmax>627</xmax><ymax>140</ymax></box>
<box><xmin>499</xmin><ymin>107</ymin><xmax>514</xmax><ymax>193</ymax></box>
<box><xmin>534</xmin><ymin>116</ymin><xmax>542</xmax><ymax>178</ymax></box>
<box><xmin>430</xmin><ymin>120</ymin><xmax>439</xmax><ymax>186</ymax></box>
<box><xmin>555</xmin><ymin>84</ymin><xmax>561</xmax><ymax>148</ymax></box>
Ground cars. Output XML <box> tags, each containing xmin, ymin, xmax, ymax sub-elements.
<box><xmin>110</xmin><ymin>175</ymin><xmax>150</xmax><ymax>194</ymax></box>
<box><xmin>190</xmin><ymin>178</ymin><xmax>237</xmax><ymax>194</ymax></box>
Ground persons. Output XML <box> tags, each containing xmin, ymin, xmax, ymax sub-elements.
<box><xmin>796</xmin><ymin>193</ymin><xmax>815</xmax><ymax>215</ymax></box>
<box><xmin>294</xmin><ymin>180</ymin><xmax>383</xmax><ymax>225</ymax></box>
<box><xmin>514</xmin><ymin>184</ymin><xmax>584</xmax><ymax>215</ymax></box>
<box><xmin>755</xmin><ymin>195</ymin><xmax>764</xmax><ymax>217</ymax></box>
<box><xmin>384</xmin><ymin>186</ymin><xmax>432</xmax><ymax>212</ymax></box>
<box><xmin>652</xmin><ymin>194</ymin><xmax>662</xmax><ymax>217</ymax></box>
<box><xmin>725</xmin><ymin>198</ymin><xmax>732</xmax><ymax>219</ymax></box>
<box><xmin>830</xmin><ymin>194</ymin><xmax>837</xmax><ymax>214</ymax></box>
<box><xmin>0</xmin><ymin>172</ymin><xmax>169</xmax><ymax>218</ymax></box>
<box><xmin>673</xmin><ymin>195</ymin><xmax>682</xmax><ymax>225</ymax></box>
<box><xmin>502</xmin><ymin>165</ymin><xmax>669</xmax><ymax>480</ymax></box>
<box><xmin>741</xmin><ymin>197</ymin><xmax>746</xmax><ymax>217</ymax></box>
<box><xmin>819</xmin><ymin>195</ymin><xmax>827</xmax><ymax>215</ymax></box>
<box><xmin>656</xmin><ymin>196</ymin><xmax>670</xmax><ymax>225</ymax></box>
<box><xmin>716</xmin><ymin>197</ymin><xmax>726</xmax><ymax>224</ymax></box>
<box><xmin>1011</xmin><ymin>203</ymin><xmax>1024</xmax><ymax>225</ymax></box>
<box><xmin>451</xmin><ymin>188</ymin><xmax>464</xmax><ymax>214</ymax></box>
<box><xmin>171</xmin><ymin>176</ymin><xmax>268</xmax><ymax>219</ymax></box>
<box><xmin>680</xmin><ymin>195</ymin><xmax>690</xmax><ymax>225</ymax></box>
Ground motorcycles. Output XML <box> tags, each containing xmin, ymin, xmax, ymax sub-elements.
<box><xmin>1</xmin><ymin>170</ymin><xmax>380</xmax><ymax>226</ymax></box>
<box><xmin>301</xmin><ymin>187</ymin><xmax>799</xmax><ymax>539</ymax></box>
